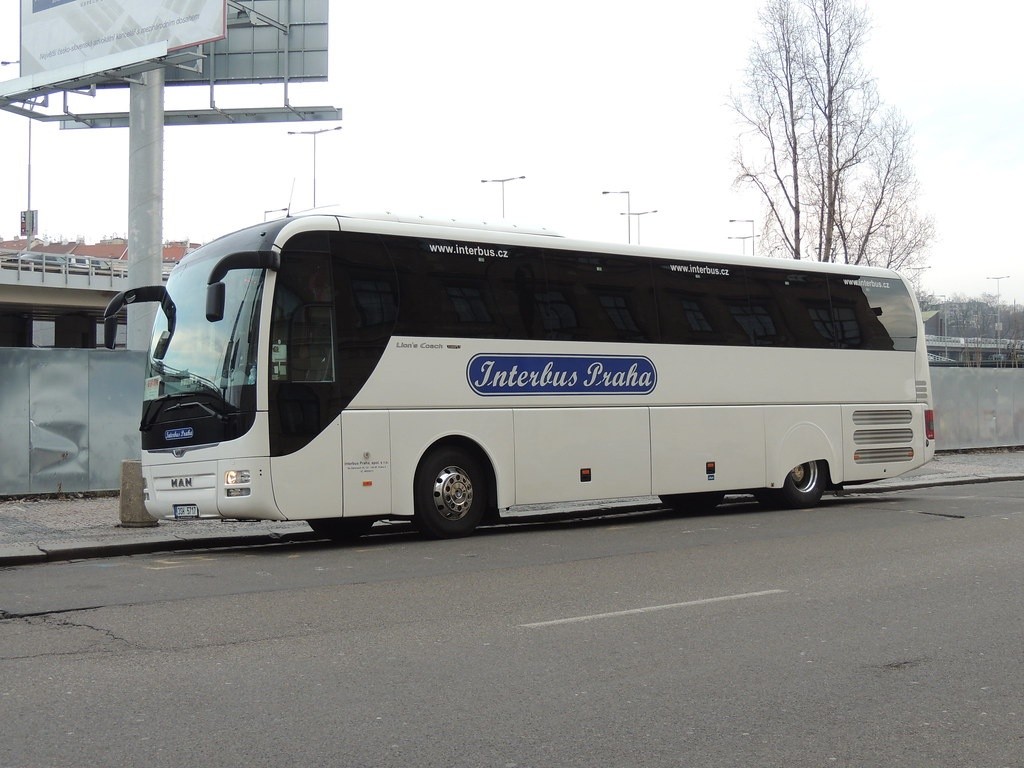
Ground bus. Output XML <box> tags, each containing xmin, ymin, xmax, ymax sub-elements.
<box><xmin>104</xmin><ymin>179</ymin><xmax>937</xmax><ymax>540</ymax></box>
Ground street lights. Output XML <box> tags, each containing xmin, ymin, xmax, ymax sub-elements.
<box><xmin>906</xmin><ymin>266</ymin><xmax>931</xmax><ymax>293</ymax></box>
<box><xmin>480</xmin><ymin>175</ymin><xmax>526</xmax><ymax>219</ymax></box>
<box><xmin>729</xmin><ymin>220</ymin><xmax>754</xmax><ymax>256</ymax></box>
<box><xmin>1</xmin><ymin>60</ymin><xmax>34</xmax><ymax>251</ymax></box>
<box><xmin>603</xmin><ymin>190</ymin><xmax>630</xmax><ymax>244</ymax></box>
<box><xmin>620</xmin><ymin>210</ymin><xmax>658</xmax><ymax>244</ymax></box>
<box><xmin>288</xmin><ymin>126</ymin><xmax>344</xmax><ymax>208</ymax></box>
<box><xmin>987</xmin><ymin>275</ymin><xmax>1010</xmax><ymax>353</ymax></box>
<box><xmin>728</xmin><ymin>234</ymin><xmax>762</xmax><ymax>254</ymax></box>
